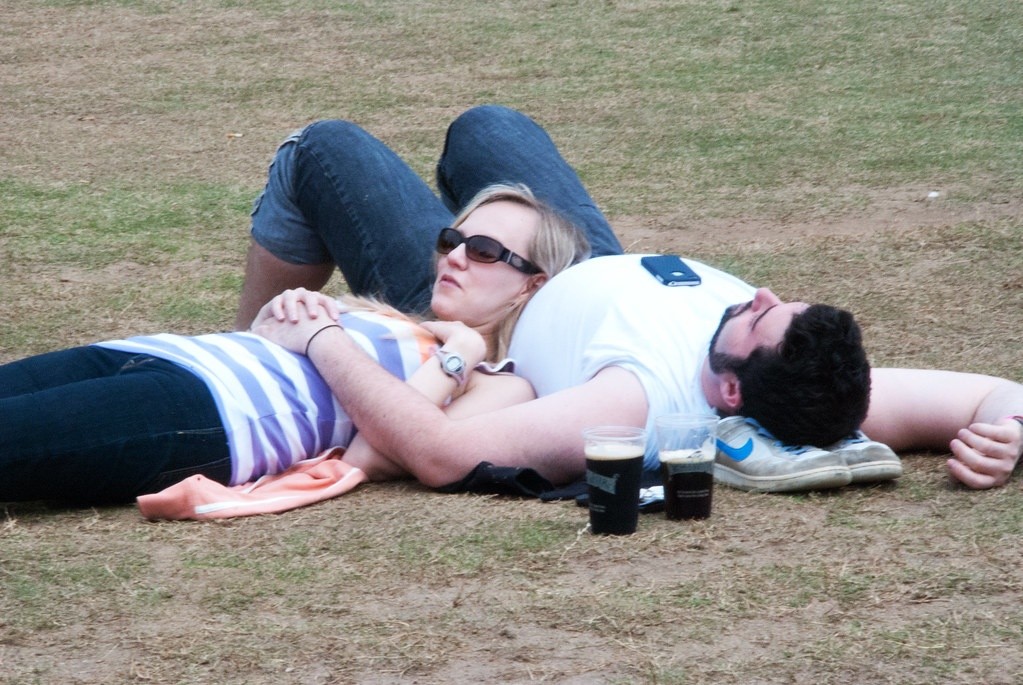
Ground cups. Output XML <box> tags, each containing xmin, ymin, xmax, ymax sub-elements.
<box><xmin>653</xmin><ymin>414</ymin><xmax>719</xmax><ymax>522</ymax></box>
<box><xmin>582</xmin><ymin>426</ymin><xmax>648</xmax><ymax>535</ymax></box>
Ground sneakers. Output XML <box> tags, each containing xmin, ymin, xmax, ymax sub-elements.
<box><xmin>685</xmin><ymin>416</ymin><xmax>852</xmax><ymax>491</ymax></box>
<box><xmin>824</xmin><ymin>428</ymin><xmax>903</xmax><ymax>480</ymax></box>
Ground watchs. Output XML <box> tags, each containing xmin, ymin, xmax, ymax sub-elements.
<box><xmin>435</xmin><ymin>350</ymin><xmax>466</xmax><ymax>386</ymax></box>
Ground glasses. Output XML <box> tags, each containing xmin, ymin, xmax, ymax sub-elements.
<box><xmin>436</xmin><ymin>227</ymin><xmax>542</xmax><ymax>277</ymax></box>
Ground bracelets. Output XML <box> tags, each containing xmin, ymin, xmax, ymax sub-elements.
<box><xmin>305</xmin><ymin>324</ymin><xmax>342</xmax><ymax>357</ymax></box>
<box><xmin>1013</xmin><ymin>416</ymin><xmax>1023</xmax><ymax>425</ymax></box>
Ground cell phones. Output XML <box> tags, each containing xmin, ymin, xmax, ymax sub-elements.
<box><xmin>639</xmin><ymin>255</ymin><xmax>701</xmax><ymax>287</ymax></box>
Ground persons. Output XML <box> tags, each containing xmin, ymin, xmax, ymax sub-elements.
<box><xmin>0</xmin><ymin>186</ymin><xmax>588</xmax><ymax>515</ymax></box>
<box><xmin>233</xmin><ymin>104</ymin><xmax>1023</xmax><ymax>488</ymax></box>
